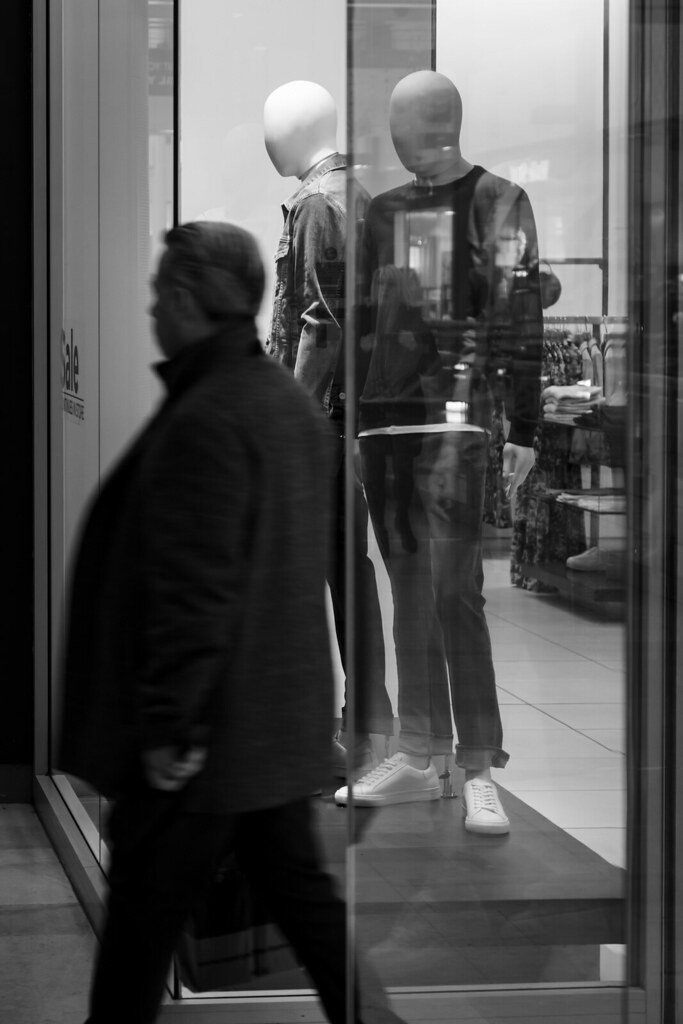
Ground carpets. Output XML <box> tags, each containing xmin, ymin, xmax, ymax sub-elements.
<box><xmin>74</xmin><ymin>712</ymin><xmax>627</xmax><ymax>995</ymax></box>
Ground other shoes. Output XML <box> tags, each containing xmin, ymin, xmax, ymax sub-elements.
<box><xmin>566</xmin><ymin>546</ymin><xmax>609</xmax><ymax>571</ymax></box>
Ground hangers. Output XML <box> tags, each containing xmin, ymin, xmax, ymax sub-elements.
<box><xmin>543</xmin><ymin>315</ymin><xmax>628</xmax><ymax>334</ymax></box>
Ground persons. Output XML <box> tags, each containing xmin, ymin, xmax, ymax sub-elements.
<box><xmin>56</xmin><ymin>219</ymin><xmax>416</xmax><ymax>1024</ymax></box>
<box><xmin>324</xmin><ymin>68</ymin><xmax>548</xmax><ymax>840</ymax></box>
<box><xmin>250</xmin><ymin>81</ymin><xmax>405</xmax><ymax>797</ymax></box>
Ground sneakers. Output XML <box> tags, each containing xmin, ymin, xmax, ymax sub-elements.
<box><xmin>460</xmin><ymin>778</ymin><xmax>510</xmax><ymax>834</ymax></box>
<box><xmin>334</xmin><ymin>752</ymin><xmax>442</xmax><ymax>806</ymax></box>
